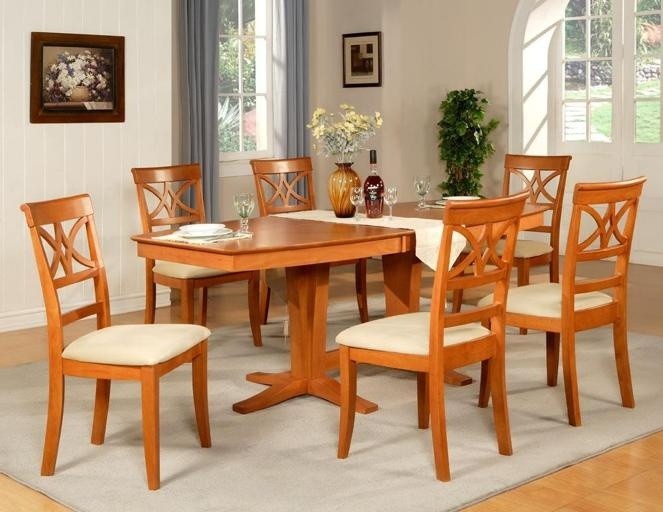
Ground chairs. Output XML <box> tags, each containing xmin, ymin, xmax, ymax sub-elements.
<box><xmin>249</xmin><ymin>152</ymin><xmax>372</xmax><ymax>328</ymax></box>
<box><xmin>129</xmin><ymin>156</ymin><xmax>266</xmax><ymax>349</ymax></box>
<box><xmin>18</xmin><ymin>190</ymin><xmax>213</xmax><ymax>494</ymax></box>
<box><xmin>452</xmin><ymin>153</ymin><xmax>577</xmax><ymax>339</ymax></box>
<box><xmin>474</xmin><ymin>171</ymin><xmax>650</xmax><ymax>429</ymax></box>
<box><xmin>331</xmin><ymin>185</ymin><xmax>537</xmax><ymax>483</ymax></box>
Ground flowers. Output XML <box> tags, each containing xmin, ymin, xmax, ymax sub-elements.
<box><xmin>304</xmin><ymin>101</ymin><xmax>385</xmax><ymax>160</ymax></box>
<box><xmin>44</xmin><ymin>48</ymin><xmax>111</xmax><ymax>103</ymax></box>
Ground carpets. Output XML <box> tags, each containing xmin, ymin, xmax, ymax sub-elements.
<box><xmin>0</xmin><ymin>291</ymin><xmax>661</xmax><ymax>512</ymax></box>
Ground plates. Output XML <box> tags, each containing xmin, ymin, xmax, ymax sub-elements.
<box><xmin>173</xmin><ymin>221</ymin><xmax>233</xmax><ymax>244</ymax></box>
<box><xmin>435</xmin><ymin>195</ymin><xmax>481</xmax><ymax>208</ymax></box>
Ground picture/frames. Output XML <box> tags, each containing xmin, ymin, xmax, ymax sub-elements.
<box><xmin>29</xmin><ymin>31</ymin><xmax>126</xmax><ymax>124</ymax></box>
<box><xmin>340</xmin><ymin>31</ymin><xmax>384</xmax><ymax>89</ymax></box>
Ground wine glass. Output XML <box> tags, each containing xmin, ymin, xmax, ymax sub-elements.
<box><xmin>347</xmin><ymin>184</ymin><xmax>367</xmax><ymax>220</ymax></box>
<box><xmin>382</xmin><ymin>184</ymin><xmax>401</xmax><ymax>221</ymax></box>
<box><xmin>410</xmin><ymin>174</ymin><xmax>432</xmax><ymax>211</ymax></box>
<box><xmin>232</xmin><ymin>190</ymin><xmax>257</xmax><ymax>237</ymax></box>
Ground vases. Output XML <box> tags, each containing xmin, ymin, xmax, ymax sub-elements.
<box><xmin>325</xmin><ymin>161</ymin><xmax>362</xmax><ymax>217</ymax></box>
<box><xmin>69</xmin><ymin>85</ymin><xmax>90</xmax><ymax>102</ymax></box>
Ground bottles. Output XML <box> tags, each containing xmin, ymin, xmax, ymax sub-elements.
<box><xmin>362</xmin><ymin>147</ymin><xmax>385</xmax><ymax>219</ymax></box>
